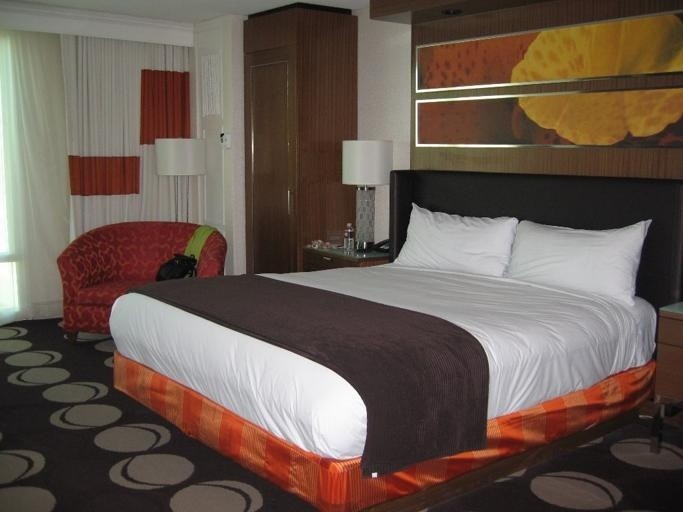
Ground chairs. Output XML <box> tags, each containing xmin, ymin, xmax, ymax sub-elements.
<box><xmin>56</xmin><ymin>220</ymin><xmax>227</xmax><ymax>341</ymax></box>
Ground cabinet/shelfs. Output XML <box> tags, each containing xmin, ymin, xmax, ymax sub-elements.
<box><xmin>243</xmin><ymin>9</ymin><xmax>357</xmax><ymax>274</ymax></box>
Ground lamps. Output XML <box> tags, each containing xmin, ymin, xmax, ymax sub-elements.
<box><xmin>340</xmin><ymin>139</ymin><xmax>392</xmax><ymax>251</ymax></box>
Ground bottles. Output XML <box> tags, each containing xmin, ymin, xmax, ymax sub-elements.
<box><xmin>343</xmin><ymin>222</ymin><xmax>355</xmax><ymax>257</ymax></box>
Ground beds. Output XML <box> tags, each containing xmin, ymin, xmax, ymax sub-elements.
<box><xmin>109</xmin><ymin>169</ymin><xmax>683</xmax><ymax>511</ymax></box>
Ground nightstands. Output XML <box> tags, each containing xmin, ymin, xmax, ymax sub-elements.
<box><xmin>651</xmin><ymin>301</ymin><xmax>683</xmax><ymax>426</ymax></box>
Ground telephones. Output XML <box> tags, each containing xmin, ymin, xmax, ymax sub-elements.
<box><xmin>364</xmin><ymin>238</ymin><xmax>389</xmax><ymax>253</ymax></box>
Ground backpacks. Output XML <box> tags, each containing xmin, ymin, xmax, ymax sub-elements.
<box><xmin>155</xmin><ymin>253</ymin><xmax>198</xmax><ymax>283</ymax></box>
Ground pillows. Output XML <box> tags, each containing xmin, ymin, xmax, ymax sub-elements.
<box><xmin>392</xmin><ymin>201</ymin><xmax>651</xmax><ymax>309</ymax></box>
